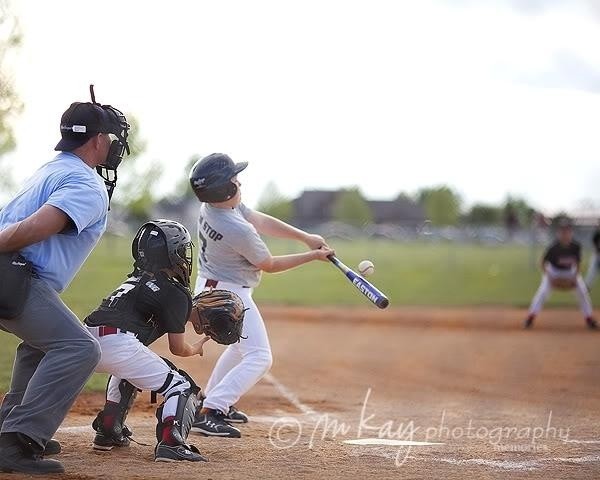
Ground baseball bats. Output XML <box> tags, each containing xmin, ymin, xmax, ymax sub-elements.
<box><xmin>318</xmin><ymin>245</ymin><xmax>389</xmax><ymax>309</ymax></box>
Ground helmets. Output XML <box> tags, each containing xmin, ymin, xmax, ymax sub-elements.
<box><xmin>132</xmin><ymin>219</ymin><xmax>195</xmax><ymax>288</ymax></box>
<box><xmin>54</xmin><ymin>102</ymin><xmax>130</xmax><ymax>172</ymax></box>
<box><xmin>190</xmin><ymin>153</ymin><xmax>248</xmax><ymax>203</ymax></box>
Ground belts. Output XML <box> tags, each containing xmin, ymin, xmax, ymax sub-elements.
<box><xmin>85</xmin><ymin>325</ymin><xmax>118</xmax><ymax>337</ymax></box>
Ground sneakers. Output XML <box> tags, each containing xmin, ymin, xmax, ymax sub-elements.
<box><xmin>191</xmin><ymin>406</ymin><xmax>248</xmax><ymax>438</ymax></box>
<box><xmin>93</xmin><ymin>430</ymin><xmax>131</xmax><ymax>451</ymax></box>
<box><xmin>154</xmin><ymin>440</ymin><xmax>210</xmax><ymax>463</ymax></box>
<box><xmin>0</xmin><ymin>431</ymin><xmax>65</xmax><ymax>474</ymax></box>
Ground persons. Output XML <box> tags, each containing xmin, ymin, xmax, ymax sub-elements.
<box><xmin>582</xmin><ymin>224</ymin><xmax>600</xmax><ymax>293</ymax></box>
<box><xmin>0</xmin><ymin>84</ymin><xmax>132</xmax><ymax>476</ymax></box>
<box><xmin>190</xmin><ymin>152</ymin><xmax>337</xmax><ymax>438</ymax></box>
<box><xmin>523</xmin><ymin>217</ymin><xmax>599</xmax><ymax>329</ymax></box>
<box><xmin>82</xmin><ymin>219</ymin><xmax>250</xmax><ymax>463</ymax></box>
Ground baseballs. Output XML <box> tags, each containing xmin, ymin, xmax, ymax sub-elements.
<box><xmin>358</xmin><ymin>261</ymin><xmax>373</xmax><ymax>276</ymax></box>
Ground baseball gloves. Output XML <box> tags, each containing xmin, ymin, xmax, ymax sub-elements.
<box><xmin>193</xmin><ymin>292</ymin><xmax>244</xmax><ymax>344</ymax></box>
